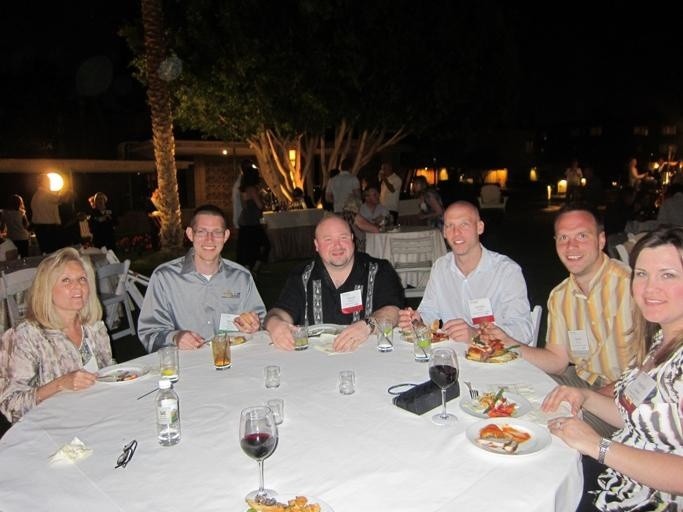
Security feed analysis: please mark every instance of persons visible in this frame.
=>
[399,200,533,345]
[540,225,683,512]
[264,212,405,352]
[0,173,115,258]
[0,247,116,422]
[137,204,267,350]
[478,204,647,439]
[564,156,683,225]
[231,159,445,251]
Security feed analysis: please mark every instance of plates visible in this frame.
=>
[463,351,520,366]
[465,417,552,455]
[95,367,145,384]
[209,333,254,348]
[307,324,340,339]
[459,389,532,418]
[241,495,333,512]
[399,329,449,346]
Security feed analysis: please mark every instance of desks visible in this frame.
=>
[263,207,324,261]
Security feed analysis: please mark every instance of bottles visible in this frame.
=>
[154,378,182,446]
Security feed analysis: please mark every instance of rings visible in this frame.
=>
[556,420,561,427]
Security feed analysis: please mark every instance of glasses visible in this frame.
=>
[192,229,225,238]
[115,440,137,470]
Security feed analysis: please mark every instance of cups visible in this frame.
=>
[376,318,393,352]
[239,406,280,502]
[290,324,308,351]
[339,371,355,395]
[264,399,284,427]
[264,365,280,389]
[157,341,179,380]
[210,335,231,371]
[412,328,431,362]
[429,347,459,426]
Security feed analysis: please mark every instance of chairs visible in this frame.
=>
[94,258,136,343]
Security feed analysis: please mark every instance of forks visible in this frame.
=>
[464,381,478,400]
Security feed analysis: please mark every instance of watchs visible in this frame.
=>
[361,317,375,334]
[597,436,612,465]
